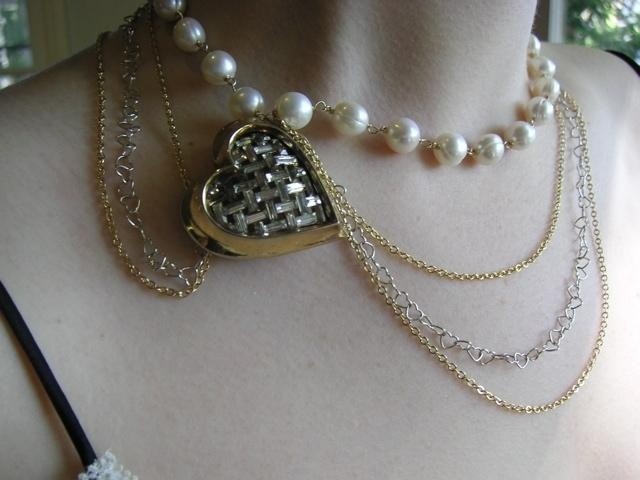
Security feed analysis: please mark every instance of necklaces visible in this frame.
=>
[95,1,610,413]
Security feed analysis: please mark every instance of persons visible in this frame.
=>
[0,0,640,480]
